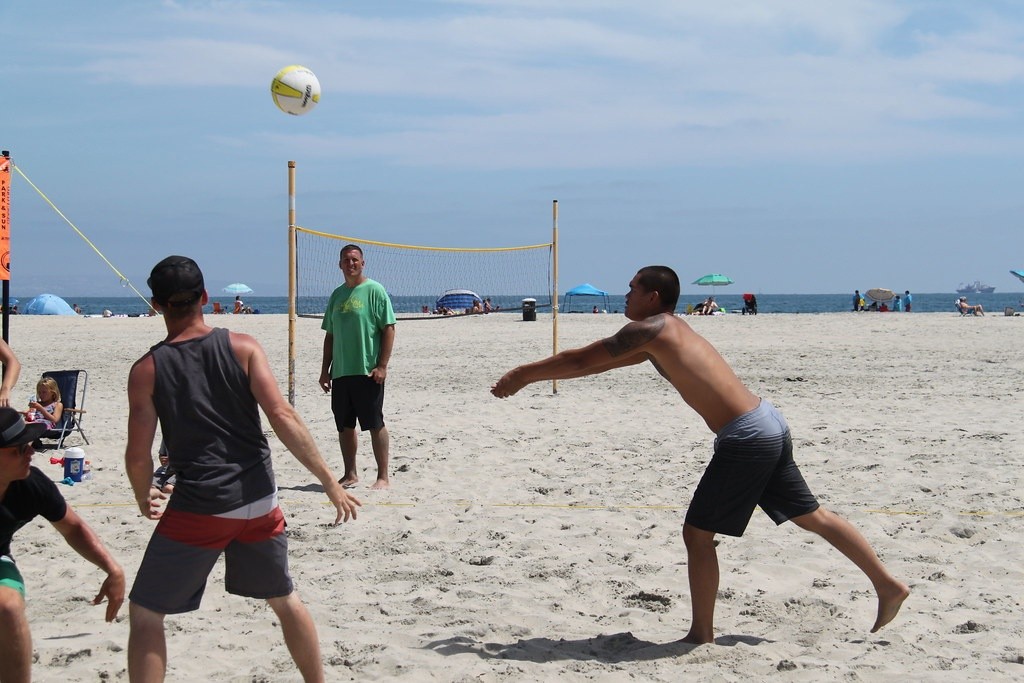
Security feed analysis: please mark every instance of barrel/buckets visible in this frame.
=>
[64,448,86,482]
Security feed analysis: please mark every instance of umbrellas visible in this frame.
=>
[1010,270,1024,282]
[865,288,896,303]
[222,283,253,296]
[691,273,734,301]
[9,297,20,306]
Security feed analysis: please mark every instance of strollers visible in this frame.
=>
[742,294,757,315]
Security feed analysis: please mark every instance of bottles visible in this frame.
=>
[83,461,90,474]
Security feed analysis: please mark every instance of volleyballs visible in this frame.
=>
[270,65,321,117]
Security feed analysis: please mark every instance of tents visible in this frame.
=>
[24,294,76,315]
[562,283,611,313]
[435,289,482,310]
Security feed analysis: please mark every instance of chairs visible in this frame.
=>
[25,370,90,450]
[213,302,224,313]
[741,293,758,315]
[422,305,428,312]
[954,301,976,317]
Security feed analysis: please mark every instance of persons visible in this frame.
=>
[0,407,125,683]
[853,290,865,311]
[319,244,396,490]
[0,305,18,314]
[960,296,983,316]
[483,298,493,314]
[893,295,902,311]
[24,377,64,449]
[73,303,80,313]
[126,256,361,683]
[0,336,21,408]
[235,296,247,313]
[593,306,598,313]
[492,266,910,644]
[471,299,483,313]
[903,290,912,311]
[700,297,718,315]
[102,307,113,317]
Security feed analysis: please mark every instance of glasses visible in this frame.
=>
[0,443,28,456]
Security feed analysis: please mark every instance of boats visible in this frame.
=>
[956,281,996,293]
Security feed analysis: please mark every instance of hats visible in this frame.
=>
[147,254,204,307]
[0,407,47,448]
